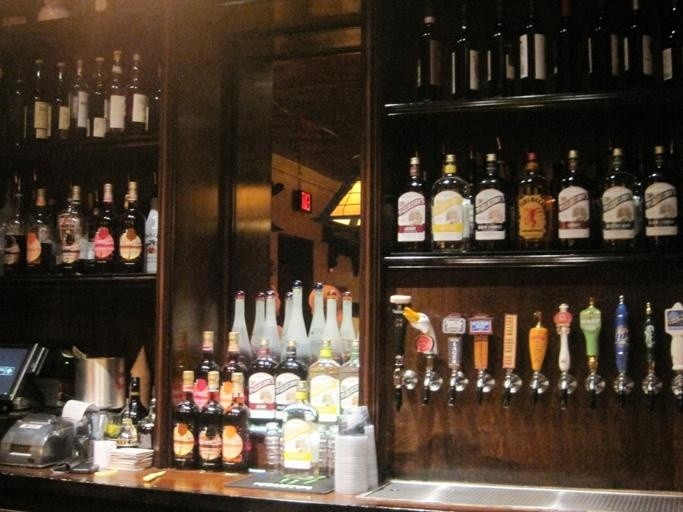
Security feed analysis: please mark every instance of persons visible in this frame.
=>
[306,283,343,329]
[267,283,284,342]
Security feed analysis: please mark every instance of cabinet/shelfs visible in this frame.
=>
[362,1,682,496]
[0,0,226,469]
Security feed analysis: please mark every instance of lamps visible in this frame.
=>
[326,176,360,228]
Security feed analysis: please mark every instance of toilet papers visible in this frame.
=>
[365,425,378,490]
[335,435,369,494]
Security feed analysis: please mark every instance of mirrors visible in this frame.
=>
[226,14,363,367]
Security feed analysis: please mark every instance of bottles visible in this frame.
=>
[221,279,358,344]
[171,371,252,472]
[2,166,158,279]
[9,43,148,146]
[115,379,157,448]
[393,145,676,253]
[191,330,357,424]
[283,381,317,478]
[402,7,676,94]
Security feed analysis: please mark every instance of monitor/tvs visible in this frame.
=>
[0,341,48,401]
